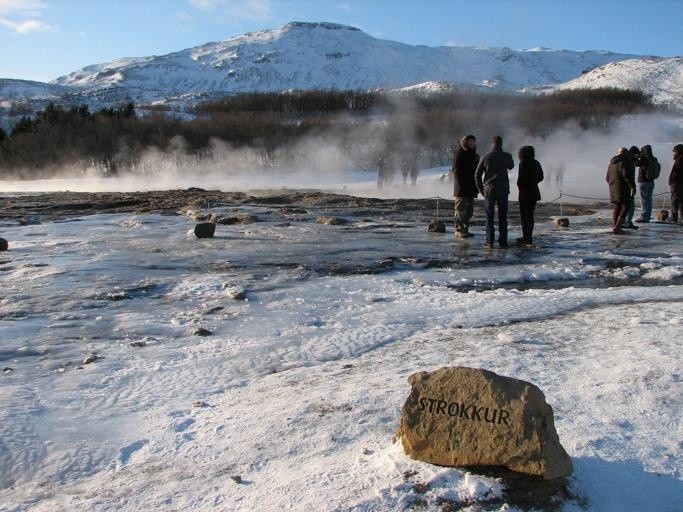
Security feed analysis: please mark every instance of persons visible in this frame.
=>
[606,144,683,234]
[452,135,543,248]
[377,155,419,190]
[548,162,565,184]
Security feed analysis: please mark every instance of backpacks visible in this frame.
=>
[643,156,660,180]
